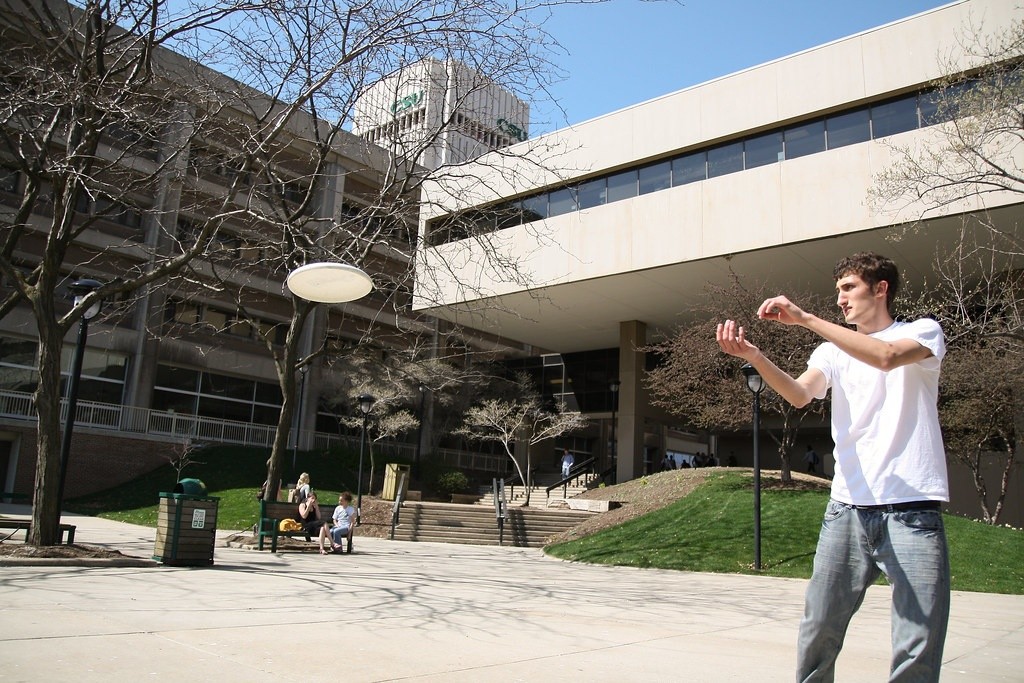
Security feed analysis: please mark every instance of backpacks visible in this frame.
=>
[288,484,307,504]
[257,482,267,502]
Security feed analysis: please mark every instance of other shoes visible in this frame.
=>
[331,542,341,549]
[332,549,343,554]
[319,549,328,555]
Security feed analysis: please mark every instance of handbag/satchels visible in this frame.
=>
[661,458,665,469]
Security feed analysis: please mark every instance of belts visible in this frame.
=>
[855,500,942,512]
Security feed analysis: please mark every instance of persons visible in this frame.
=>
[715,251,951,683]
[295,472,312,542]
[258,457,283,503]
[801,444,822,473]
[298,489,342,555]
[659,451,737,471]
[329,493,357,553]
[560,446,575,480]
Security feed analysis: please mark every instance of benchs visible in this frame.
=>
[451,494,483,503]
[0,519,77,545]
[257,499,354,553]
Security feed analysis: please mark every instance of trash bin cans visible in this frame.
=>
[151,478,221,568]
[382,463,411,502]
